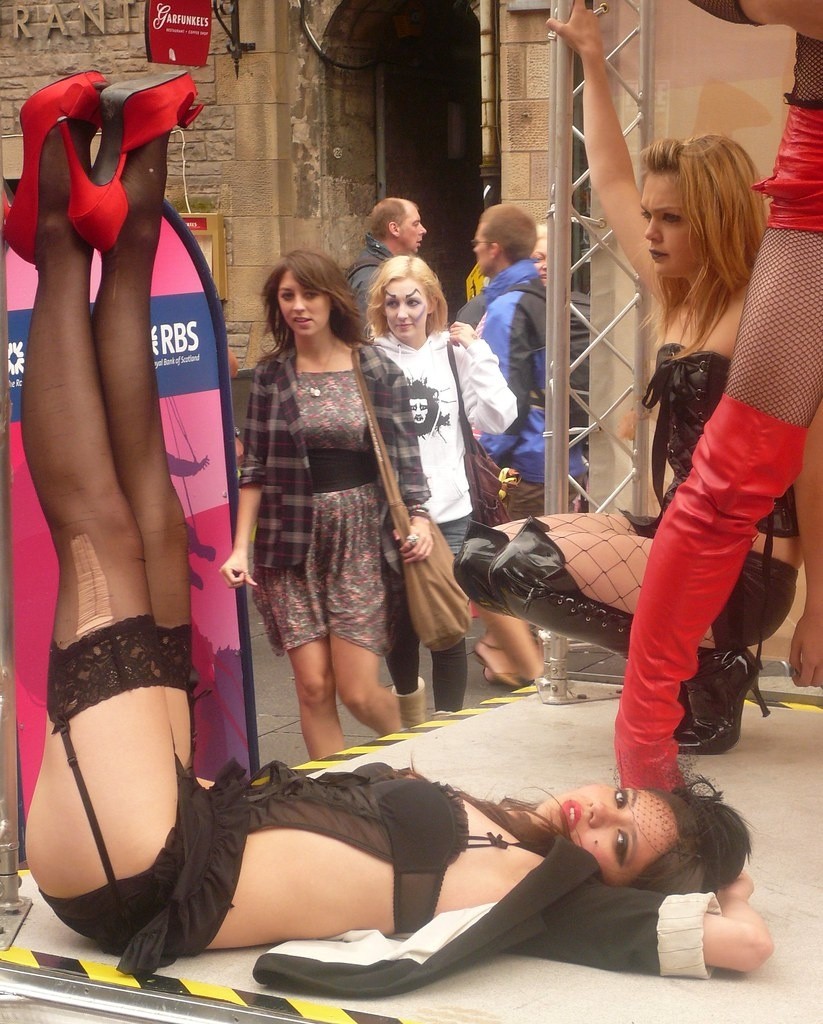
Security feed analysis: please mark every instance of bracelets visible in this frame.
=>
[408,504,431,521]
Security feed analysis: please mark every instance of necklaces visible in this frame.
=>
[299,338,336,398]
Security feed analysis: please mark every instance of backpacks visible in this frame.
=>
[497,281,590,456]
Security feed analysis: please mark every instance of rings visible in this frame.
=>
[789,669,801,677]
[406,534,419,545]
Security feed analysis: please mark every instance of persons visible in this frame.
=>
[453,0,823,754]
[344,197,426,337]
[455,204,590,688]
[455,225,547,330]
[613,0,823,799]
[218,250,434,761]
[367,254,519,727]
[0,71,773,1001]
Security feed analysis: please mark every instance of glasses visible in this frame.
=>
[471,240,499,247]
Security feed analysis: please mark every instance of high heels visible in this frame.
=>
[0,72,110,265]
[58,70,204,251]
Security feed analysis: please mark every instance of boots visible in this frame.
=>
[393,676,426,731]
[488,515,769,755]
[451,519,691,734]
[614,394,808,793]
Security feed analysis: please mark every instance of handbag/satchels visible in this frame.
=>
[463,439,520,527]
[400,513,471,652]
